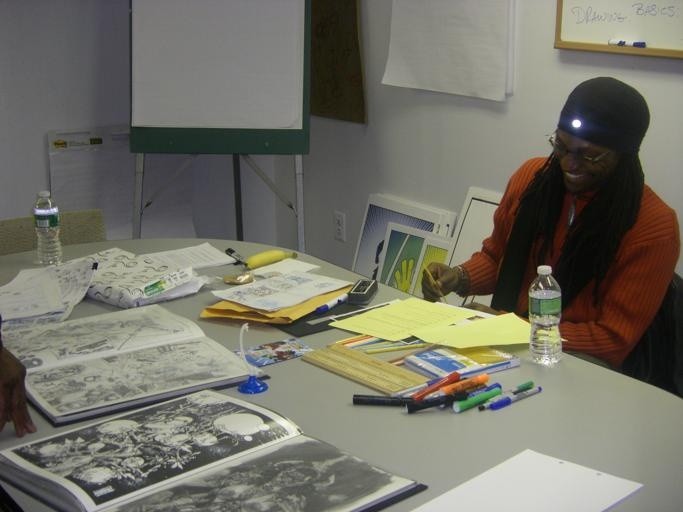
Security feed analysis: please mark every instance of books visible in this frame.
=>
[0,304,253,427]
[0,390,429,512]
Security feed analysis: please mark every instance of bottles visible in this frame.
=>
[32,191,62,265]
[527,265,563,365]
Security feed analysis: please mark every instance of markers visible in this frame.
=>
[225,248,247,265]
[316,292,350,314]
[608,38,645,48]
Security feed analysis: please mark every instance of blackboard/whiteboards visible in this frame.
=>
[129,0,311,154]
[554,0,683,61]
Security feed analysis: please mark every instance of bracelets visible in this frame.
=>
[455,265,467,295]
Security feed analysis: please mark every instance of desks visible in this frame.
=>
[0,236,682,512]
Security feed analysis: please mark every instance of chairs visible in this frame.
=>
[619,270,682,398]
[0,208,108,257]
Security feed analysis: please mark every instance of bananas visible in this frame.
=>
[243,249,298,270]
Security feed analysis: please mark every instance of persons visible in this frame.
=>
[0,315,37,439]
[420,75,681,373]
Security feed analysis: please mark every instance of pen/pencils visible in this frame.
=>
[424,267,449,304]
[353,371,542,414]
[365,343,428,354]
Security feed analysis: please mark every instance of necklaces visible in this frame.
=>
[565,198,578,232]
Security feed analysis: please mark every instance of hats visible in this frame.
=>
[556,77,650,151]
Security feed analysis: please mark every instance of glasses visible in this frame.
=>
[546,131,608,163]
